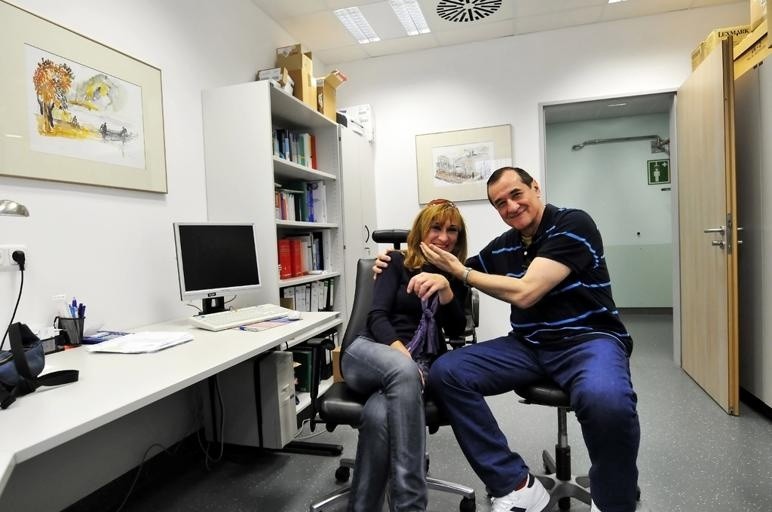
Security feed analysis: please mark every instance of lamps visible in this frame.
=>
[0,199,29,217]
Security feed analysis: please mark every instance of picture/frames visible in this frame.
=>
[415,124,513,206]
[0,0,168,195]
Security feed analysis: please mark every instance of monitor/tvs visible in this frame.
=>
[172,220,264,315]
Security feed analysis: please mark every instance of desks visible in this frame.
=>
[1,300,338,495]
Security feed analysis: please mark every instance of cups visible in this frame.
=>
[53,316,86,346]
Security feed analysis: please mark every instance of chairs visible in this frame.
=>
[310,230,476,512]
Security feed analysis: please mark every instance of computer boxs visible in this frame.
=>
[200,349,298,450]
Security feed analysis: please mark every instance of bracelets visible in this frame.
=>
[463,267,472,288]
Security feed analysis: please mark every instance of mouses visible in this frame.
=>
[285,309,303,321]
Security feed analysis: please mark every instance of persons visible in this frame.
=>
[373,167,640,512]
[340,198,467,512]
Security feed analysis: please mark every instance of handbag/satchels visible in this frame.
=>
[0,322,79,409]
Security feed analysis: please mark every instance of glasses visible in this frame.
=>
[428,199,456,207]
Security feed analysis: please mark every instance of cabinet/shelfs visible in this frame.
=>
[202,81,347,416]
[338,125,377,325]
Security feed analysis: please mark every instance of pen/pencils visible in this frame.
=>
[78,302,83,317]
[68,304,73,318]
[72,297,78,319]
[81,306,85,318]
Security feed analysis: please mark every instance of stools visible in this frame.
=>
[515,386,592,512]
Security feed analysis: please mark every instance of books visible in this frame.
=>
[272,129,339,380]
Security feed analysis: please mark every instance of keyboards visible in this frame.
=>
[187,302,292,333]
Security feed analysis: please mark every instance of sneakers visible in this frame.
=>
[490,471,550,512]
[590,498,601,512]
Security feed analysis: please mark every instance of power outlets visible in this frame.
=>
[0,245,30,272]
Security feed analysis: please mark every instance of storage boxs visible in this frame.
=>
[692,0,767,75]
[332,346,345,383]
[275,43,347,122]
